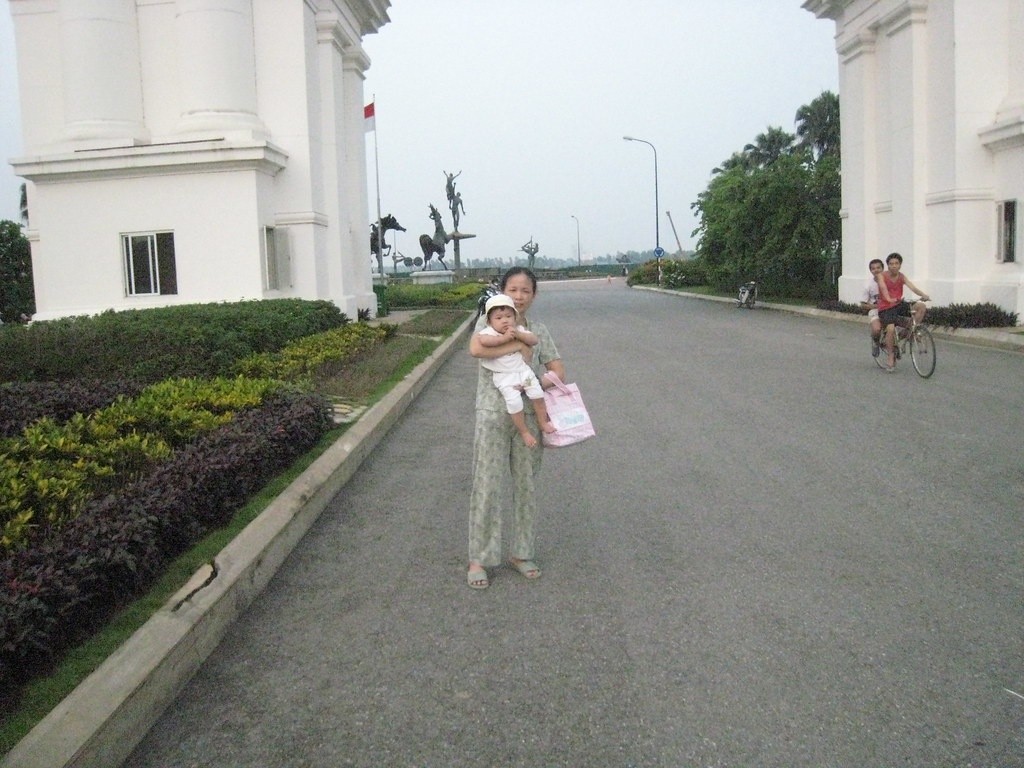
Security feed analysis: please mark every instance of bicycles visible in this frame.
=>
[874,296,937,379]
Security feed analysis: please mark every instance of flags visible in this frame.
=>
[364,102,375,132]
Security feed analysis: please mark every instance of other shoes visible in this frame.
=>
[888,353,896,368]
[898,329,915,342]
[871,336,880,357]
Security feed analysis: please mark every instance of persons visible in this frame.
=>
[878,252,929,372]
[443,170,465,232]
[859,258,900,360]
[392,253,404,263]
[469,266,565,590]
[478,295,557,448]
[522,242,539,268]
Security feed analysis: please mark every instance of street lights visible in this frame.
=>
[623,135,660,288]
[571,215,580,270]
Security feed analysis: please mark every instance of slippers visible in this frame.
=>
[467,566,489,589]
[506,558,542,579]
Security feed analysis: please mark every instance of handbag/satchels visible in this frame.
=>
[540,371,595,448]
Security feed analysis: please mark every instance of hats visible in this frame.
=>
[485,295,520,325]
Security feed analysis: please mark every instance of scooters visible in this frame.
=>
[736,274,763,310]
